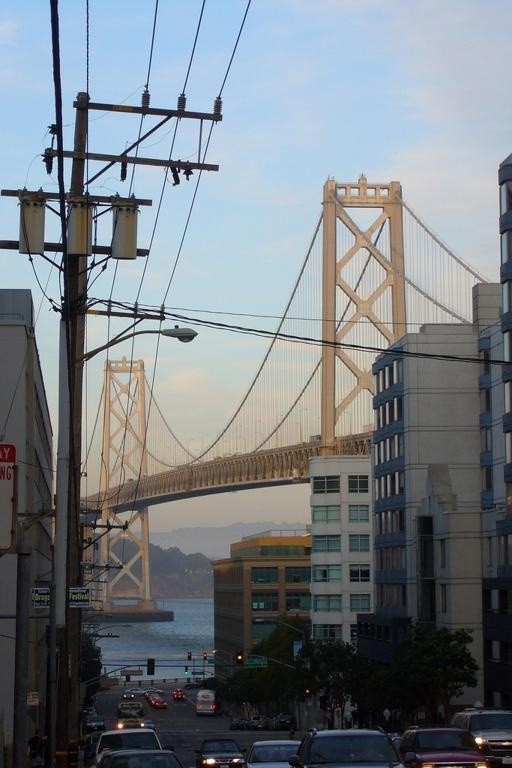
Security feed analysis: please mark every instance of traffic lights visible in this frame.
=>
[184,651,243,672]
[147,658,155,675]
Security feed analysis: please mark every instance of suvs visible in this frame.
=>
[450,709,512,766]
[289,725,405,768]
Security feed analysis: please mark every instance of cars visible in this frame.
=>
[245,739,302,768]
[117,683,217,729]
[95,727,183,768]
[193,738,248,768]
[399,727,491,768]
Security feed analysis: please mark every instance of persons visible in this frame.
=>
[381,705,391,720]
[438,701,446,727]
[27,727,41,757]
